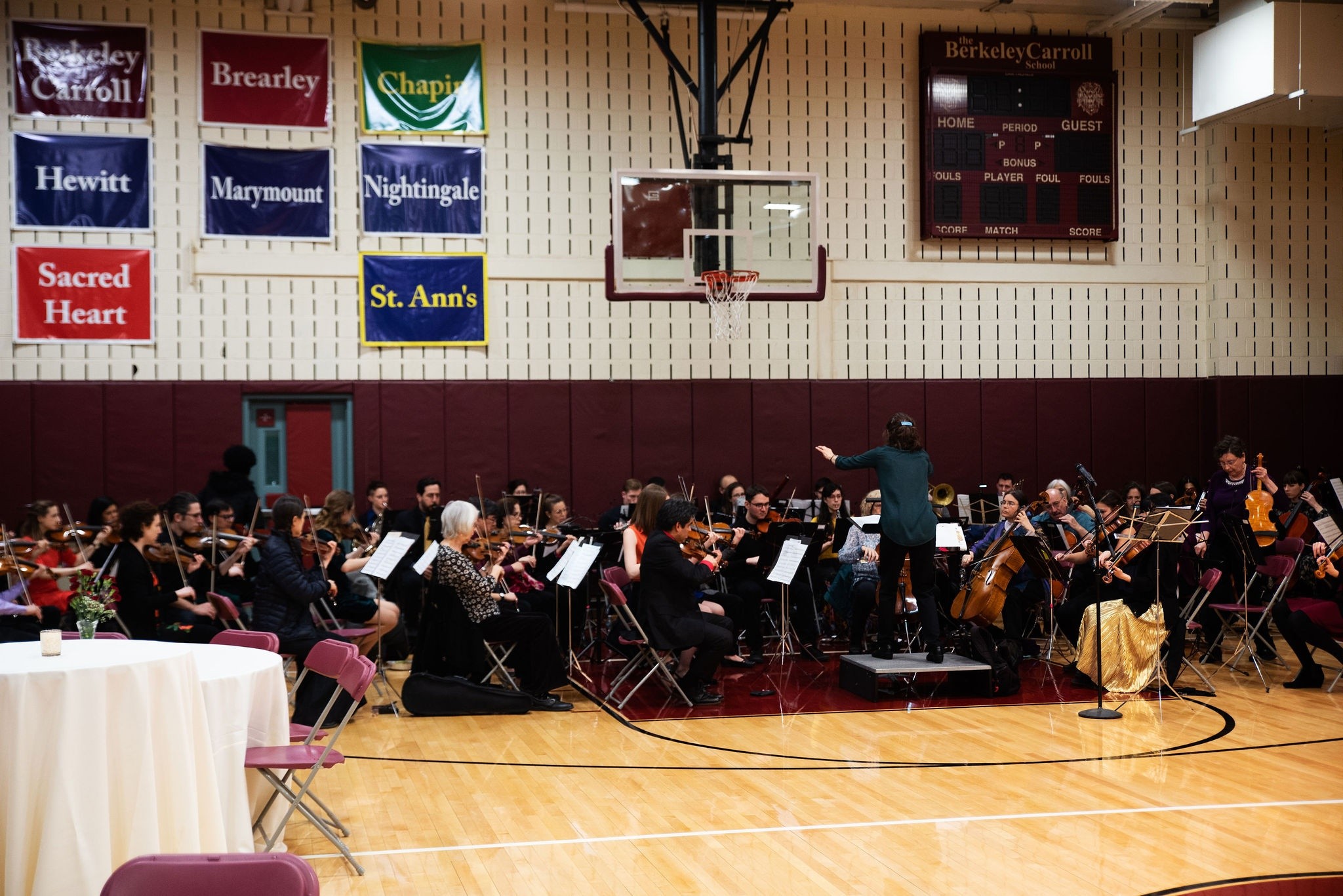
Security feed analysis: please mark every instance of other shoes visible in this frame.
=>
[720,655,755,668]
[707,679,717,684]
[1070,676,1092,685]
[992,667,1021,697]
[751,647,762,663]
[800,645,828,660]
[1248,648,1279,661]
[848,629,862,654]
[318,721,337,728]
[1063,661,1079,672]
[1200,651,1222,664]
[1283,664,1324,688]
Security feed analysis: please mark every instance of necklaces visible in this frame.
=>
[1225,478,1244,486]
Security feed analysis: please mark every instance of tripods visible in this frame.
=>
[1201,520,1270,696]
[1111,507,1194,724]
[343,523,1076,707]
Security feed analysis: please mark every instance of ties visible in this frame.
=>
[423,517,432,553]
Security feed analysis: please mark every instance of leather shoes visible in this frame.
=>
[519,688,573,711]
[672,683,725,704]
[927,646,943,663]
[872,644,893,660]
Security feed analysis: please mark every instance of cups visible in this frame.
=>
[40,629,62,656]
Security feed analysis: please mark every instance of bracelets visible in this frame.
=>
[830,455,835,462]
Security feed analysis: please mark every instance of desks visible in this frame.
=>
[0,637,297,896]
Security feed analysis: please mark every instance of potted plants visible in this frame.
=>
[69,567,118,638]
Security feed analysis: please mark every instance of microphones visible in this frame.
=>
[1075,462,1098,487]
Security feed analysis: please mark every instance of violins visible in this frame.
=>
[0,554,62,582]
[334,523,372,544]
[1244,453,1277,547]
[297,534,341,557]
[756,511,803,533]
[688,521,761,543]
[1102,541,1154,584]
[184,526,264,551]
[0,538,69,555]
[519,524,568,546]
[466,537,515,560]
[488,527,538,544]
[46,520,123,543]
[1086,518,1127,559]
[1314,539,1343,579]
[144,542,218,571]
[680,539,728,568]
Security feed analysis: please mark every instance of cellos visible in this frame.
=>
[950,491,1050,624]
[875,542,920,655]
[1037,491,1085,604]
[1276,472,1323,538]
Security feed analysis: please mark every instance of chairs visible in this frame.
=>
[1255,535,1304,636]
[599,565,668,687]
[1201,554,1295,695]
[208,590,294,683]
[287,636,360,743]
[1149,566,1222,692]
[210,623,280,648]
[245,655,383,876]
[311,597,377,647]
[99,850,322,896]
[1031,548,1077,661]
[738,597,792,657]
[480,638,522,694]
[600,577,693,710]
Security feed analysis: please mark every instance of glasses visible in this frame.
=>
[485,518,496,524]
[550,506,567,515]
[1219,456,1239,466]
[217,514,234,519]
[510,514,523,520]
[1001,501,1019,507]
[181,513,200,518]
[1045,495,1064,510]
[749,503,770,509]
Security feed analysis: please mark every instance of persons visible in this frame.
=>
[435,498,574,712]
[1192,433,1343,688]
[598,478,756,704]
[198,443,266,530]
[20,498,120,633]
[928,472,1100,696]
[0,563,49,643]
[807,477,882,655]
[816,412,947,664]
[144,490,262,631]
[312,488,400,657]
[1053,479,1224,692]
[461,480,592,670]
[114,500,222,643]
[254,496,351,729]
[358,479,446,654]
[704,474,831,665]
[86,497,124,573]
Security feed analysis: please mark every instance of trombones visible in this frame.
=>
[926,481,955,518]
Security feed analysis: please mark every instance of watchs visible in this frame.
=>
[91,543,99,550]
